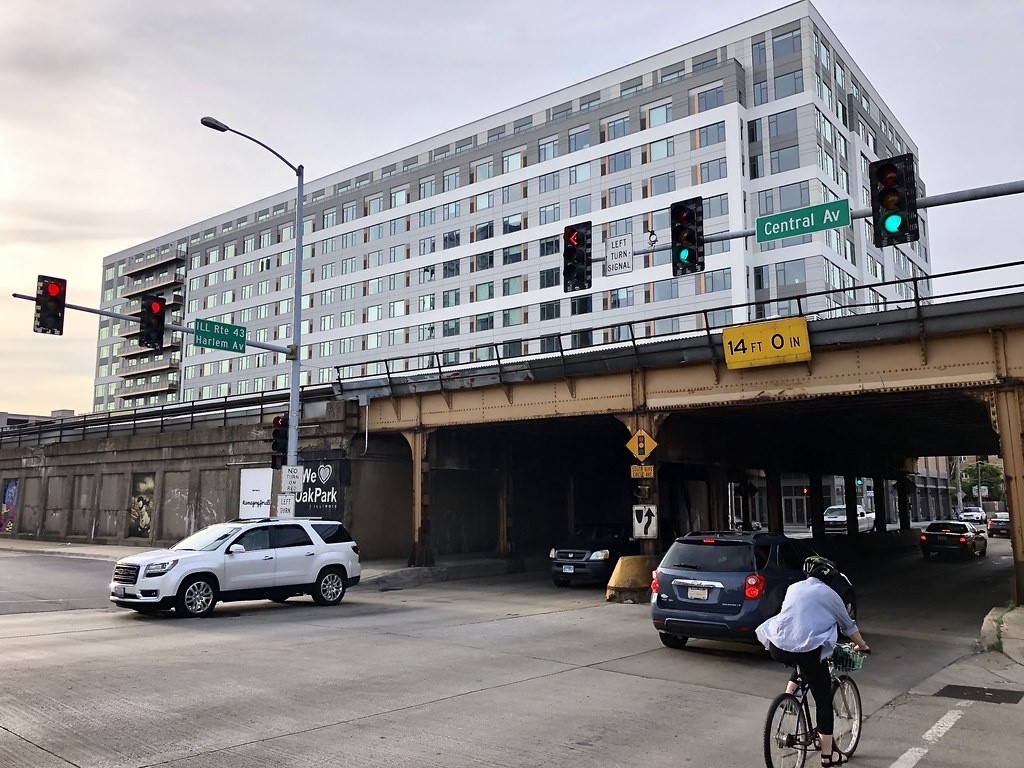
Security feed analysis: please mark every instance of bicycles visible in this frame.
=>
[763,641,872,768]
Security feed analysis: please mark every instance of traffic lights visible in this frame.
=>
[671,195,705,278]
[270,411,288,470]
[803,489,808,494]
[139,294,166,350]
[563,219,594,293]
[857,477,862,486]
[32,274,67,337]
[868,152,920,249]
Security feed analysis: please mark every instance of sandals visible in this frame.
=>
[779,699,796,716]
[821,751,849,767]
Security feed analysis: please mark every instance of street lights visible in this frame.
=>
[198,118,304,516]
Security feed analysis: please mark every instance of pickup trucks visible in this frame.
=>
[823,504,877,537]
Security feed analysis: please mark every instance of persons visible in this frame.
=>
[755,557,869,768]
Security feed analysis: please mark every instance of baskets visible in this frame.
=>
[831,644,867,674]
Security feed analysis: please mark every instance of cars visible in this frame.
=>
[920,520,987,562]
[958,507,987,525]
[986,512,1011,538]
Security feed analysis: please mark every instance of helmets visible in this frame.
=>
[802,555,836,579]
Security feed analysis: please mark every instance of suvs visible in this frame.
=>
[548,517,679,588]
[107,517,362,618]
[651,528,852,649]
